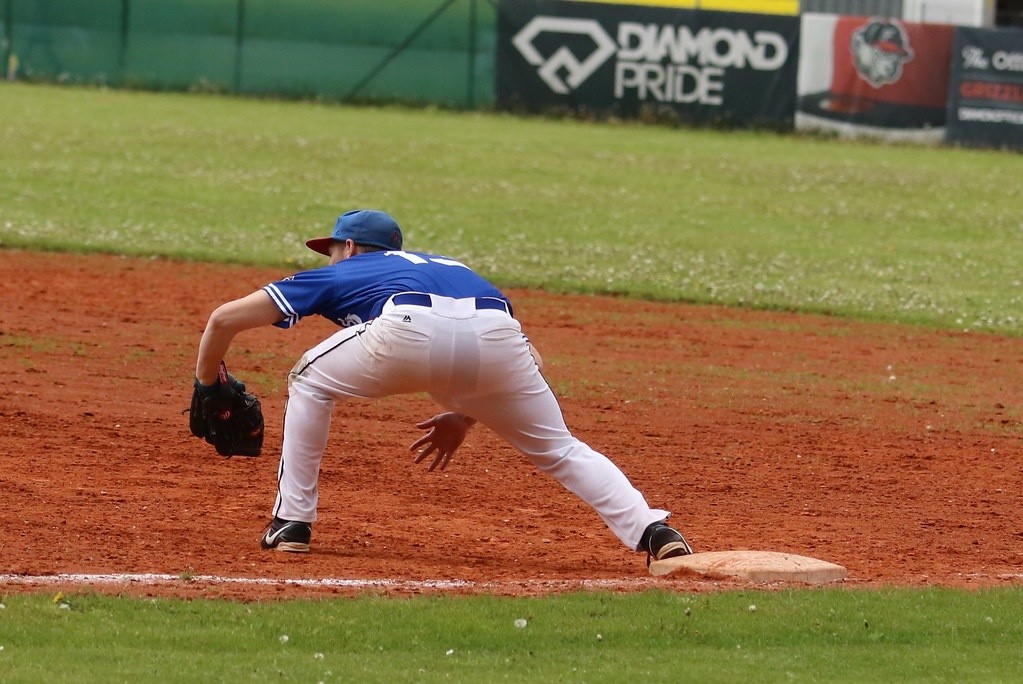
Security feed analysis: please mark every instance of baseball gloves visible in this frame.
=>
[190,375,265,457]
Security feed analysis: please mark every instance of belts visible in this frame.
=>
[392,293,506,311]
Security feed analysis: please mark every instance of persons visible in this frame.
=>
[189,209,693,559]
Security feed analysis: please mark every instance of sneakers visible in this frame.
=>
[255,517,312,552]
[640,517,692,568]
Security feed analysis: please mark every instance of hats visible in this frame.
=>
[305,210,403,256]
